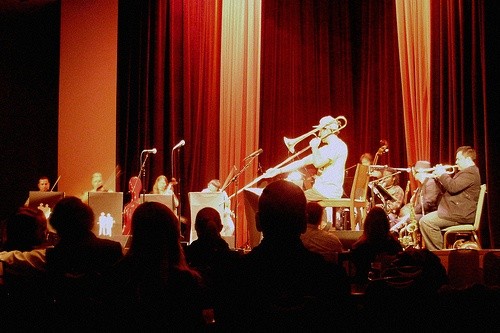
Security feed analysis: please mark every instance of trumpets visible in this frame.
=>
[411,165,459,178]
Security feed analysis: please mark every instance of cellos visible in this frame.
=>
[368,140,389,176]
[166,178,178,195]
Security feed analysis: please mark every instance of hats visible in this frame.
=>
[415,161,432,168]
[49,197,94,230]
[312,115,338,130]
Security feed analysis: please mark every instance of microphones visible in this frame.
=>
[173,139,186,151]
[244,148,264,161]
[142,148,157,154]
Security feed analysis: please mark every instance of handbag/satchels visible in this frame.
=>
[367,246,448,290]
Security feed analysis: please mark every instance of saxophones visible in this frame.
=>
[406,186,420,246]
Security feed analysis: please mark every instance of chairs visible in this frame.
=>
[440,183,487,251]
[317,163,370,232]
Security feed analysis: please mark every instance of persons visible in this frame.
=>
[81,171,113,205]
[262,115,348,205]
[200,179,235,236]
[389,160,441,234]
[381,167,403,225]
[403,145,481,251]
[24,177,51,207]
[360,153,374,165]
[0,181,499,333]
[149,175,179,215]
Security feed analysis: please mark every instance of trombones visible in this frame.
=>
[218,116,347,207]
